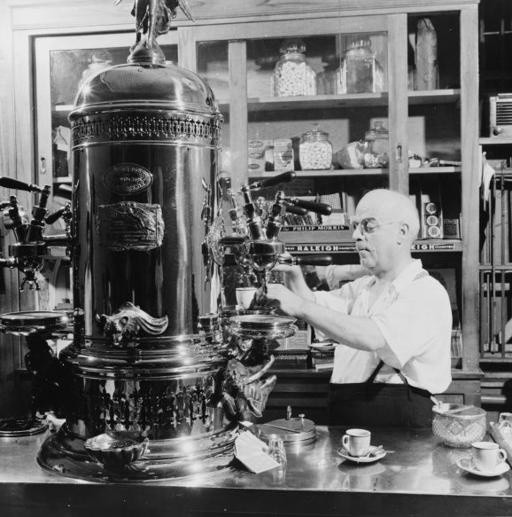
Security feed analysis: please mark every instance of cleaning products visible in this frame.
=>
[415,18,436,93]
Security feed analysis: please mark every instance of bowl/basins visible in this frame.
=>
[431,403,488,449]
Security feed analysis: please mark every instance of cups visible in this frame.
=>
[471,442,508,471]
[235,288,258,311]
[341,429,373,457]
[266,438,287,468]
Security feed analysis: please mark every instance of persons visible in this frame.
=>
[255,188,453,428]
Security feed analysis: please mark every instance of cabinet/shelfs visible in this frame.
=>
[0,0,512,423]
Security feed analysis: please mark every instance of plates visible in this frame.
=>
[337,445,388,463]
[455,456,511,478]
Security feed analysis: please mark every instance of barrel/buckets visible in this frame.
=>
[0,309,73,416]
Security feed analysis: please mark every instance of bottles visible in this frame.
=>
[271,37,317,98]
[360,120,390,169]
[297,121,334,172]
[332,36,387,96]
